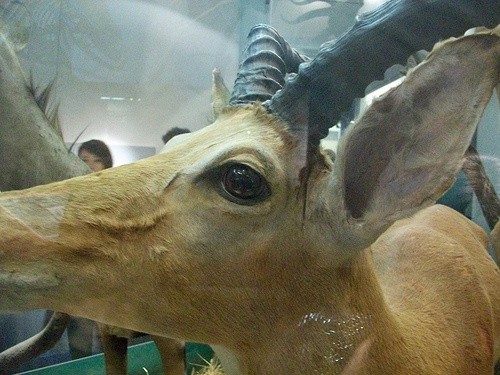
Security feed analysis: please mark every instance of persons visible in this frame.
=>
[65,131,123,358]
[92,317,191,373]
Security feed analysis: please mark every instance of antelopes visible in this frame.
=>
[1,0,500,375]
